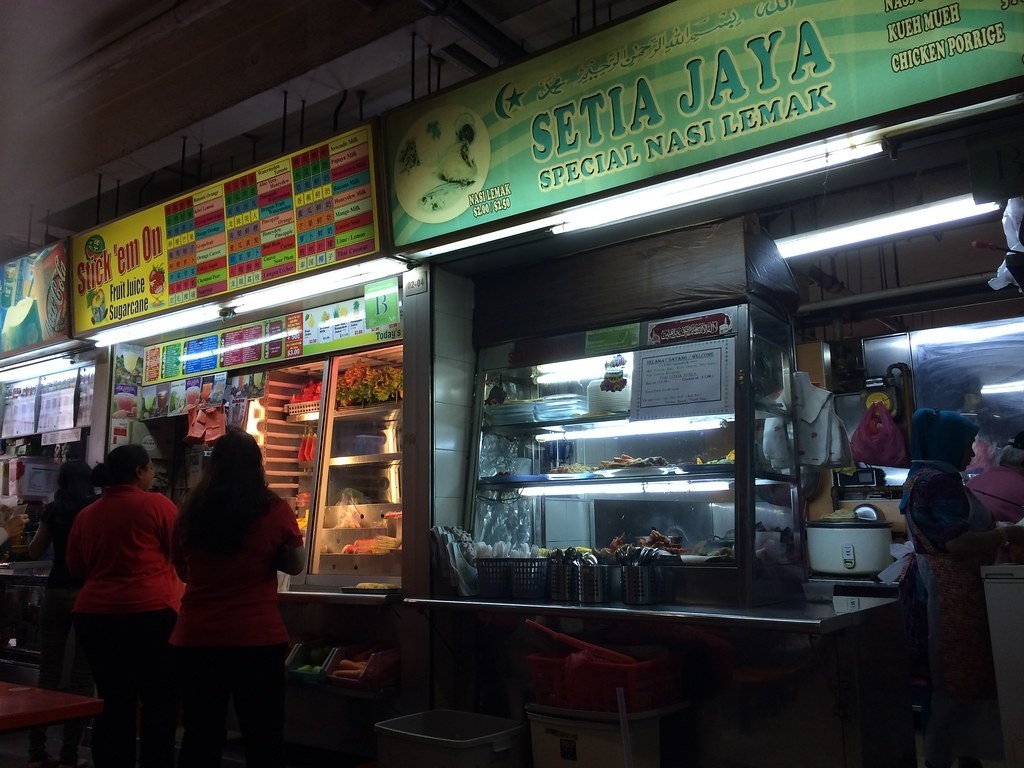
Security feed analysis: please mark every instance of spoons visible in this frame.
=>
[555,546,660,564]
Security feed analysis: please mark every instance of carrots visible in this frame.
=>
[332,659,367,679]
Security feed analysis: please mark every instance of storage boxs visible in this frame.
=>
[284,634,399,759]
[527,712,661,768]
[374,707,526,768]
[526,650,676,712]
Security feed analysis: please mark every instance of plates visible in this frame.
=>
[592,465,676,478]
[480,474,546,484]
[533,392,585,422]
[393,104,491,223]
[585,378,632,415]
[678,462,735,474]
[483,400,533,426]
[545,471,593,481]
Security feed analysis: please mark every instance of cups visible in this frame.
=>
[117,392,134,410]
[187,373,262,403]
[158,391,168,410]
[124,353,138,371]
[144,395,153,410]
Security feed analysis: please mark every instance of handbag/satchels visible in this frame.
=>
[851,402,910,468]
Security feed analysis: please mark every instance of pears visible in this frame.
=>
[296,665,322,672]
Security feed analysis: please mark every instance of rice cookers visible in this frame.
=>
[805,503,896,576]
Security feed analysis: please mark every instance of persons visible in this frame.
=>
[28,459,96,768]
[898,408,1024,768]
[169,429,304,768]
[0,504,30,546]
[67,444,184,768]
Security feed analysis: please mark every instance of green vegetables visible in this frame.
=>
[337,358,404,407]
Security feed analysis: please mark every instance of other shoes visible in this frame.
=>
[25,755,61,768]
[58,758,89,768]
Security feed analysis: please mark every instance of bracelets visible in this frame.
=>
[995,527,1010,548]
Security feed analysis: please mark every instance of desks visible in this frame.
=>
[0,681,104,734]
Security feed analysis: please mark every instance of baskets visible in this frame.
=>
[473,557,550,598]
[526,647,685,713]
